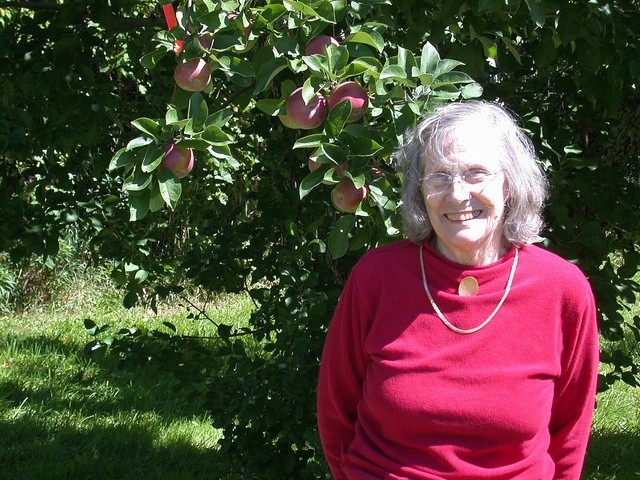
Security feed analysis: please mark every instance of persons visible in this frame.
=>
[316,97,600,480]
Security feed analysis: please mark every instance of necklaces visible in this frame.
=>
[419,244,519,334]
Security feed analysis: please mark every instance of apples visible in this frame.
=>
[156,142,195,179]
[362,168,386,208]
[174,57,211,91]
[286,86,328,128]
[308,147,350,185]
[304,35,340,59]
[330,178,367,214]
[194,32,219,57]
[225,12,251,40]
[327,81,369,123]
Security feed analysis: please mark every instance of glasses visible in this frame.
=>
[418,168,504,191]
